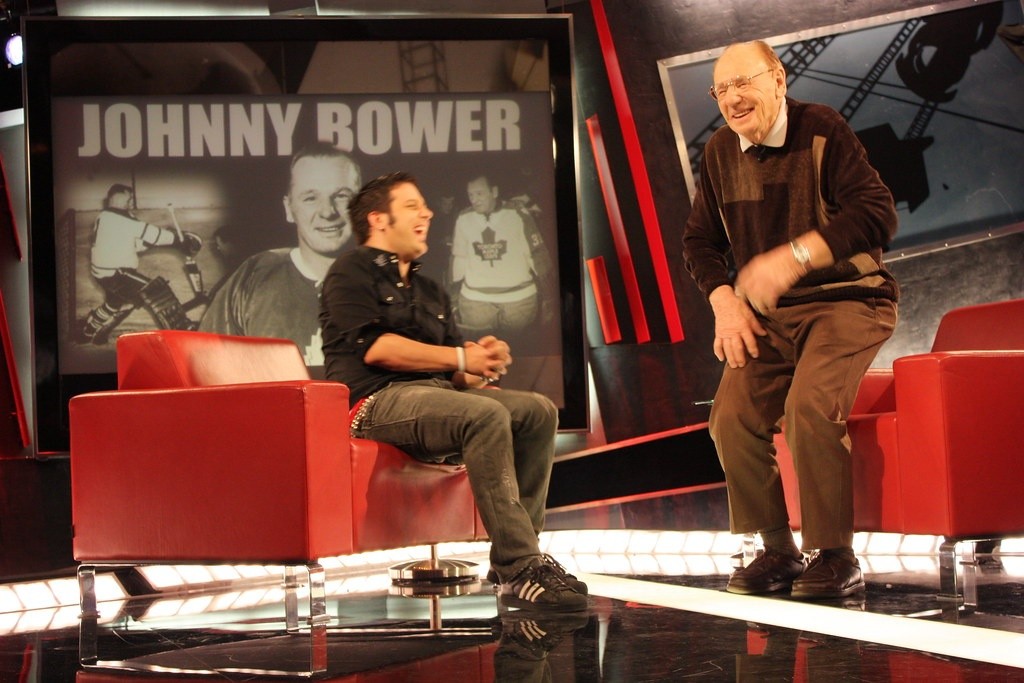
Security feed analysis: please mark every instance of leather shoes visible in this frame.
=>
[791,554,866,598]
[727,554,806,593]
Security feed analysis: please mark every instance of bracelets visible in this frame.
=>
[456,346,465,372]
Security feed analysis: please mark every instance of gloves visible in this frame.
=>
[175,231,202,256]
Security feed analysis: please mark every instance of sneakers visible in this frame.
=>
[501,557,588,612]
[546,561,587,595]
[502,609,588,650]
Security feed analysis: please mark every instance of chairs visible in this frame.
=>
[72,330,496,683]
[746,299,1023,603]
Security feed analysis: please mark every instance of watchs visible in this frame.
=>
[791,239,813,274]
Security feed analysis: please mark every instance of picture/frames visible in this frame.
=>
[17,11,592,457]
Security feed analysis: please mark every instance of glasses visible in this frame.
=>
[708,69,774,101]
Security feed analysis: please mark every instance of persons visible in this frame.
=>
[448,176,553,330]
[492,613,589,683]
[315,172,589,610]
[213,224,234,259]
[682,40,900,599]
[198,142,362,367]
[78,183,200,345]
